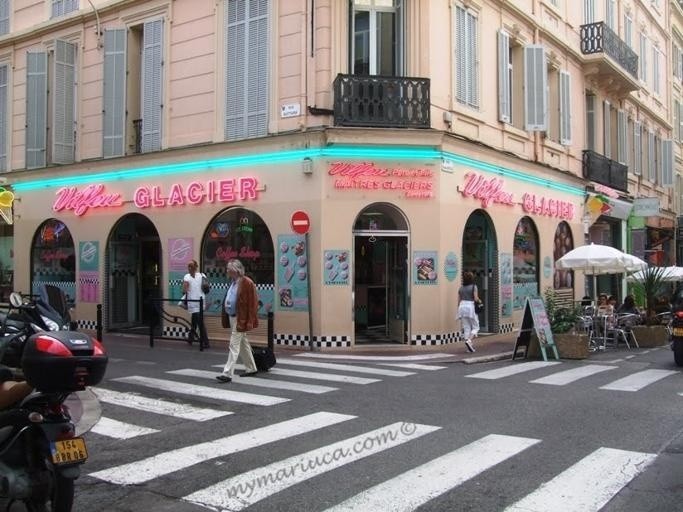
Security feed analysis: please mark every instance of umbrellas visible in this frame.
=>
[553,241,633,304]
[624,265,680,283]
[664,265,682,285]
[583,250,647,305]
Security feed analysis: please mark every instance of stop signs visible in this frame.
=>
[291,210,310,234]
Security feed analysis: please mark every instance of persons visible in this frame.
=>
[215,258,260,384]
[582,295,645,343]
[456,270,482,354]
[180,259,213,352]
[668,281,683,342]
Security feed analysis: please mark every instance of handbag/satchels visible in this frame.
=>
[201,276,210,293]
[475,302,485,313]
[178,294,187,310]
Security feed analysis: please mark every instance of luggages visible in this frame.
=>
[253,348,276,371]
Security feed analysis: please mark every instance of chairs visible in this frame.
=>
[572,304,640,352]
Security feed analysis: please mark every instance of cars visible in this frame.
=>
[667,281,683,365]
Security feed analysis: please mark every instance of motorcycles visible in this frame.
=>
[0,290,111,512]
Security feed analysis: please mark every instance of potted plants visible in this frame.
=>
[543,288,590,359]
[624,259,675,347]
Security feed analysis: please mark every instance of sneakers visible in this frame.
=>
[240,372,257,376]
[216,376,232,382]
[465,339,476,352]
